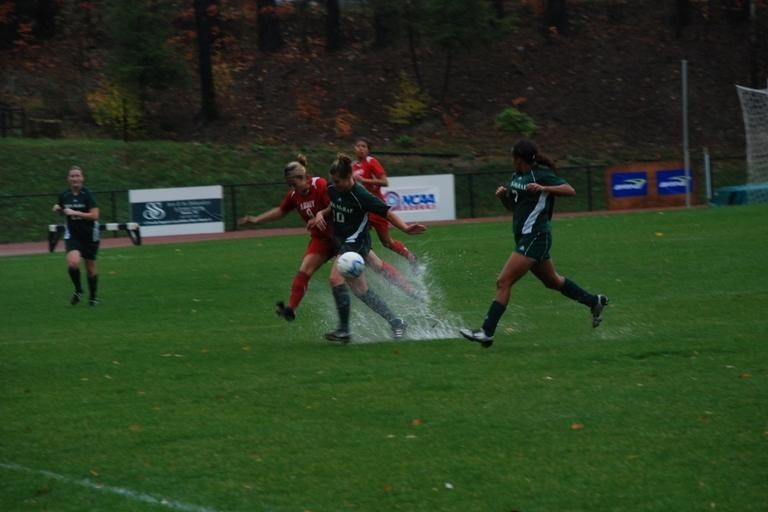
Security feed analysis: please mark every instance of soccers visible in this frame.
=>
[337,252,364,278]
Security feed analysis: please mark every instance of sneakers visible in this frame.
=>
[591,294,609,328]
[391,319,408,341]
[325,328,350,341]
[89,296,98,305]
[459,327,495,348]
[275,301,295,321]
[71,291,82,305]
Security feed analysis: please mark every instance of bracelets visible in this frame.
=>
[539,184,545,192]
[256,215,260,222]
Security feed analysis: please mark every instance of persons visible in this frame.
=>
[52,166,103,307]
[238,154,430,320]
[314,154,426,343]
[460,139,609,347]
[347,138,421,276]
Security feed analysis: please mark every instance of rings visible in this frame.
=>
[318,220,323,223]
